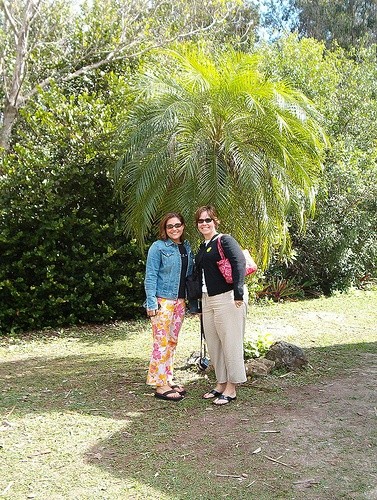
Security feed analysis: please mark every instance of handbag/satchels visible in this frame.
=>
[217,235,257,284]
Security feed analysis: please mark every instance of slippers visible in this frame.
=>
[202,390,223,399]
[154,390,183,401]
[212,395,237,405]
[170,384,186,395]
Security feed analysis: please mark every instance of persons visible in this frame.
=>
[145,212,198,400]
[194,205,257,405]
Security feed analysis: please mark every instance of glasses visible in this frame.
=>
[197,218,213,223]
[166,222,183,229]
